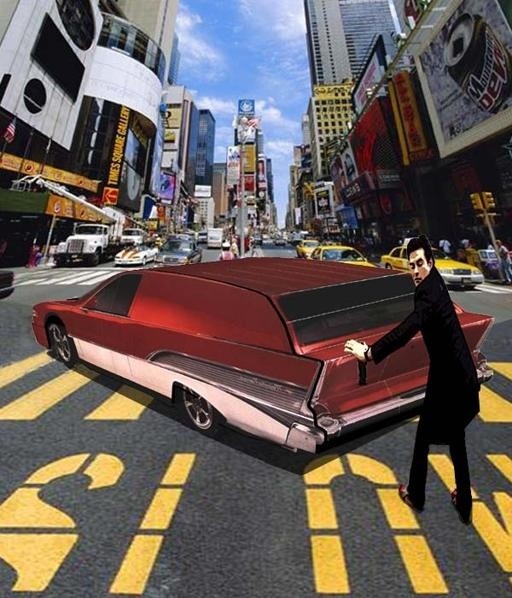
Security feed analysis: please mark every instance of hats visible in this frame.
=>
[222,241,230,247]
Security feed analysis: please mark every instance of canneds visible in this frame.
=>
[443,11,512,115]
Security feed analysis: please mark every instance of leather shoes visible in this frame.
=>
[399,484,425,512]
[451,488,471,525]
